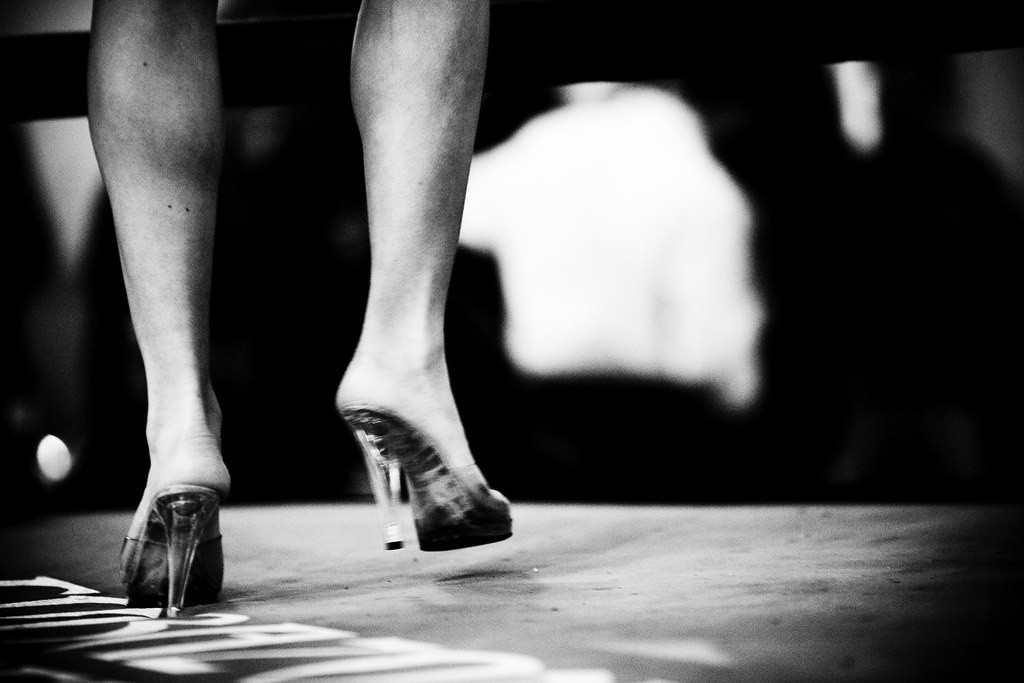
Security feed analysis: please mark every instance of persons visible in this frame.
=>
[87,0,513,616]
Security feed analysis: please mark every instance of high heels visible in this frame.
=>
[342,405,515,556]
[119,485,226,616]
